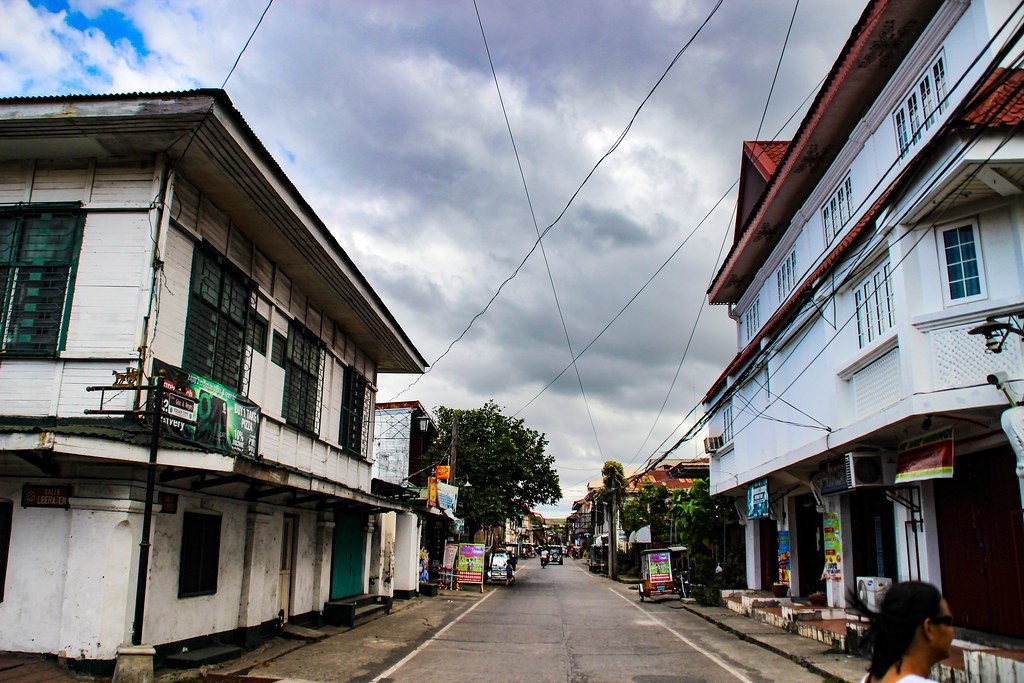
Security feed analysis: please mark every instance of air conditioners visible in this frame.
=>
[857,576,892,613]
[845,449,905,489]
[704,436,724,454]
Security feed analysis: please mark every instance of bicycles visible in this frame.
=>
[542,561,546,569]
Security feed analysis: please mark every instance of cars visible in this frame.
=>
[547,545,563,565]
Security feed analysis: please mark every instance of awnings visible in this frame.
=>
[629,525,652,543]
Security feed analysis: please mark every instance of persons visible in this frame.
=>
[522,545,537,560]
[540,548,549,566]
[564,547,578,559]
[505,560,514,587]
[484,556,491,585]
[845,581,955,683]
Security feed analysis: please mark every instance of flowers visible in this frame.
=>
[772,581,786,585]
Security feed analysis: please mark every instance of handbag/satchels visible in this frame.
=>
[716,563,722,573]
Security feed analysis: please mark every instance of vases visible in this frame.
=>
[808,595,827,607]
[772,585,788,597]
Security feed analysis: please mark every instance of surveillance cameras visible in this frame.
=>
[987,371,1010,385]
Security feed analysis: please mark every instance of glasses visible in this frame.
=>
[927,616,953,627]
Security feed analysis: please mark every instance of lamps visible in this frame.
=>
[417,414,430,431]
